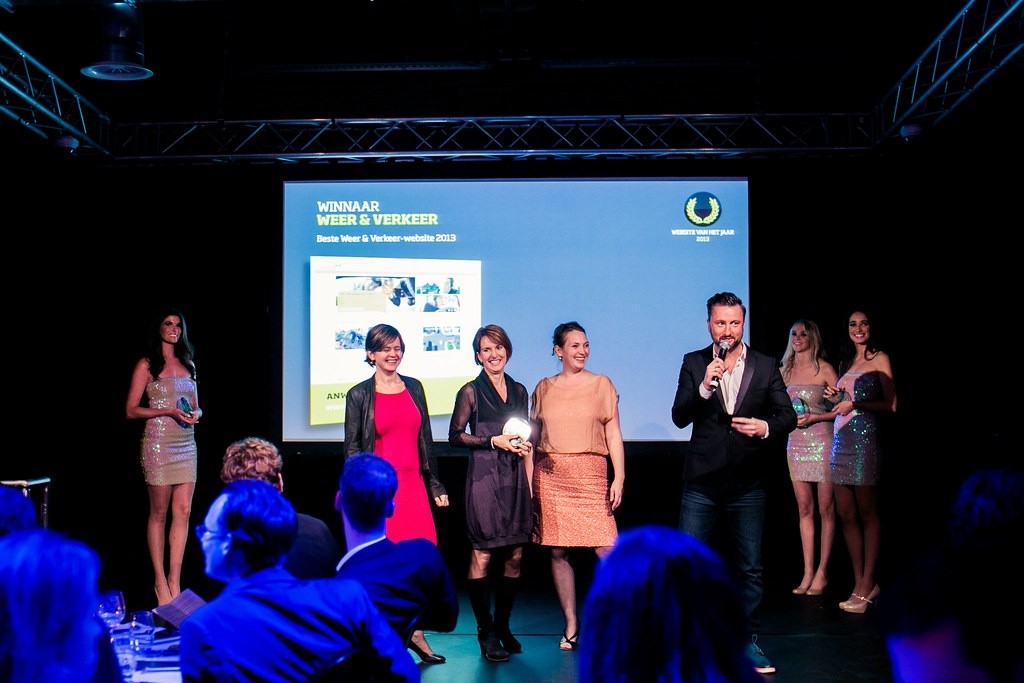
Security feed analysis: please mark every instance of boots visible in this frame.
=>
[494,575,522,652]
[469,575,509,661]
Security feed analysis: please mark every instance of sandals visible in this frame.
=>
[560,624,577,651]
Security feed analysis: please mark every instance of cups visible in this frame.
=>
[131,611,155,651]
[110,636,137,683]
[99,591,126,628]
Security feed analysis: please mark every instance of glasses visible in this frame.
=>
[196,525,224,540]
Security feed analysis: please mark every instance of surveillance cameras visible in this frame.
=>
[899,125,921,143]
[57,137,80,154]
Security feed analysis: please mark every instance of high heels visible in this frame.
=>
[838,584,880,614]
[410,639,446,663]
[793,577,829,596]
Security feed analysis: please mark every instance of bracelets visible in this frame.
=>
[490,436,496,450]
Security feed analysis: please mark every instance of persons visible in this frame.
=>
[447,324,533,662]
[573,524,757,683]
[822,307,898,614]
[887,463,1024,683]
[779,318,840,596]
[344,322,450,663]
[671,292,799,674]
[218,437,336,580]
[0,487,127,683]
[333,453,459,641]
[125,308,203,607]
[179,480,422,683]
[0,529,106,683]
[523,321,625,651]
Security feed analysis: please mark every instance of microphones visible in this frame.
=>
[709,340,731,388]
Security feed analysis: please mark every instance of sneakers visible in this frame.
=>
[746,642,776,673]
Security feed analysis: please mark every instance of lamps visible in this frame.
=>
[79,0,153,81]
